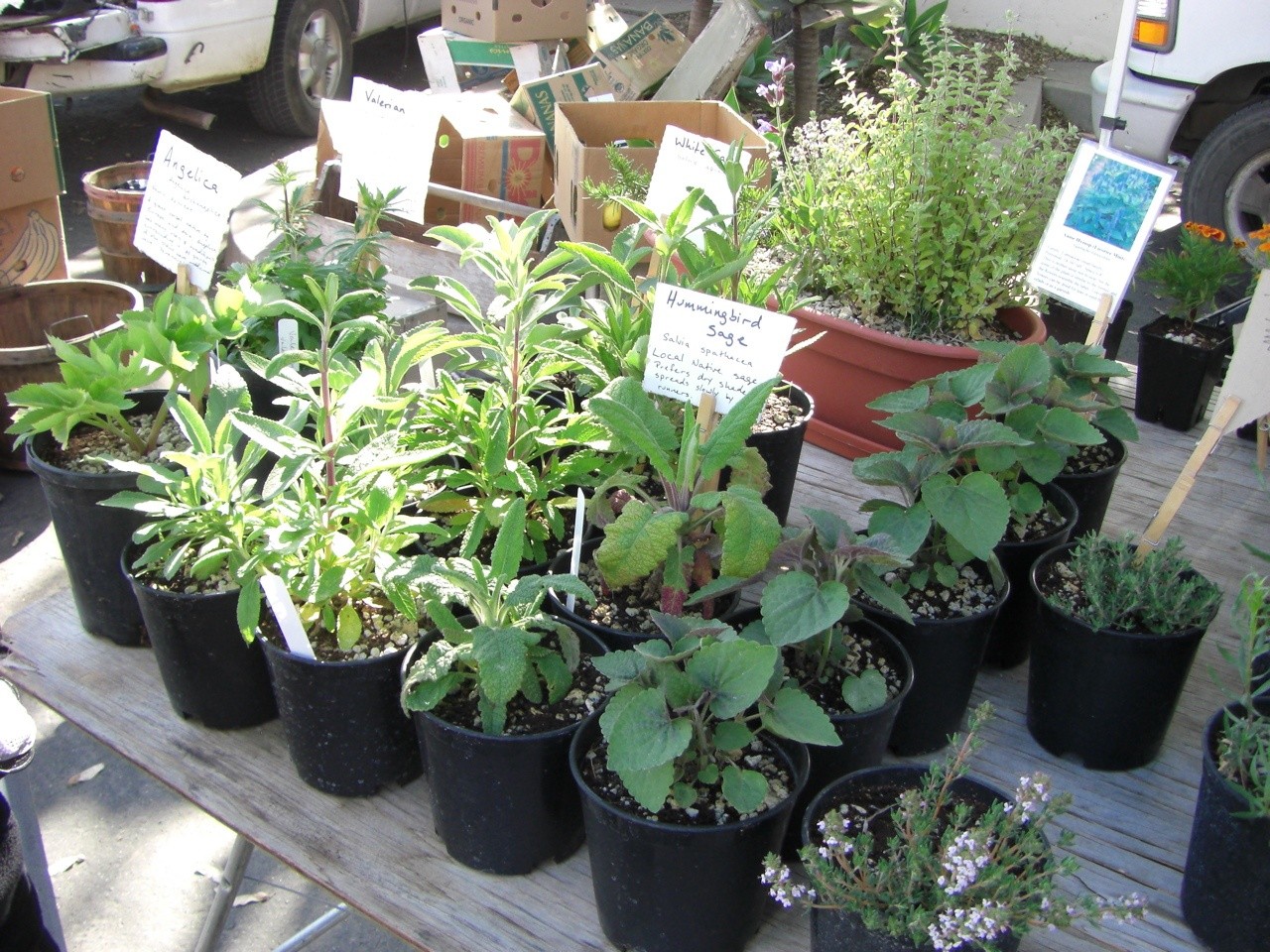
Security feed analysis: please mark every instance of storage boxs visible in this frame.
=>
[317,1,770,262]
[0,195,71,288]
[0,86,67,210]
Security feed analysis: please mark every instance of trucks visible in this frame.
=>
[1083,1,1268,308]
[1,0,442,140]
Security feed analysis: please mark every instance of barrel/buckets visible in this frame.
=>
[79,161,176,293]
[0,278,144,471]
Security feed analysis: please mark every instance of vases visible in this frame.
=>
[802,764,1054,951]
[1135,315,1232,432]
[642,225,1048,463]
[1041,297,1134,387]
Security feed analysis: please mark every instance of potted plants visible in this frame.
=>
[4,133,1269,952]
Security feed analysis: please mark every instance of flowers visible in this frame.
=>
[755,8,1080,343]
[758,700,1148,952]
[1245,223,1270,298]
[1137,223,1247,336]
